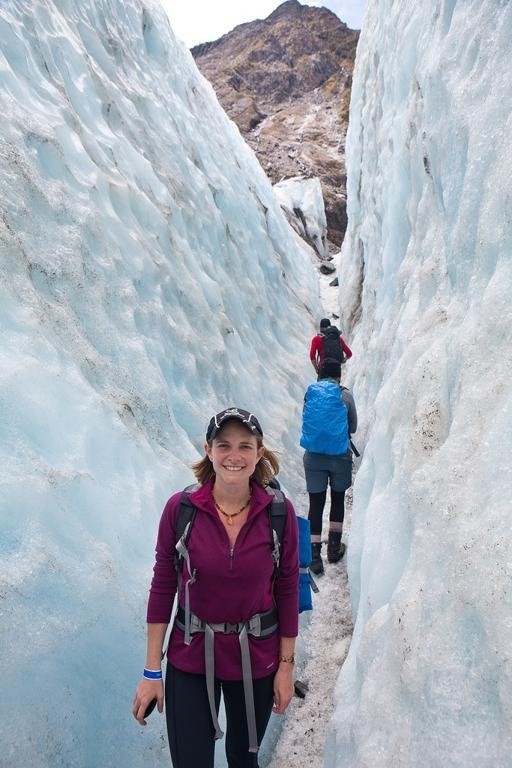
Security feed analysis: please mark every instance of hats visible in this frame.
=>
[205,407,265,449]
[319,317,332,332]
[319,357,342,378]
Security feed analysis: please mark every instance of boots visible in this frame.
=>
[309,540,325,576]
[326,530,346,563]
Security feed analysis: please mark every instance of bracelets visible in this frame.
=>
[140,665,164,682]
[277,652,297,666]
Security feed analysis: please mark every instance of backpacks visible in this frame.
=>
[298,379,351,457]
[317,329,345,363]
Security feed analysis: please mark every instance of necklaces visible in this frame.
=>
[209,494,256,526]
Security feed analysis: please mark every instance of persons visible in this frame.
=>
[128,403,305,768]
[308,317,355,386]
[296,357,359,578]
